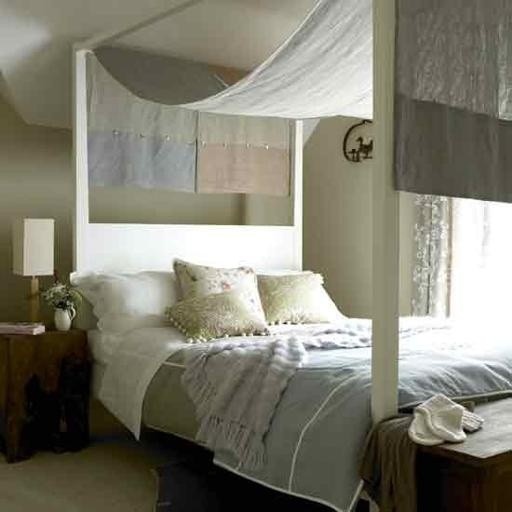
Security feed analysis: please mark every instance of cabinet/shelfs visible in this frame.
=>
[415,396,512,512]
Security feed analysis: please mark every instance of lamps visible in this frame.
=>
[12,218,54,322]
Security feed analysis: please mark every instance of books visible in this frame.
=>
[1,321,48,336]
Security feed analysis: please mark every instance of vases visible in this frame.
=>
[51,308,76,332]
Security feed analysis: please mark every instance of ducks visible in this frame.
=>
[356,137,373,158]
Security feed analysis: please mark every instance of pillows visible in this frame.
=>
[71,261,351,346]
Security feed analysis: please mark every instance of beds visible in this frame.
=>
[72,0,512,512]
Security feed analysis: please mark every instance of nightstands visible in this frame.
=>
[0,327,88,464]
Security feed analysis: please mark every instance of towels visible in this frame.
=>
[360,400,474,512]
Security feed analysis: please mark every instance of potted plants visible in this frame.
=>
[41,279,86,305]
